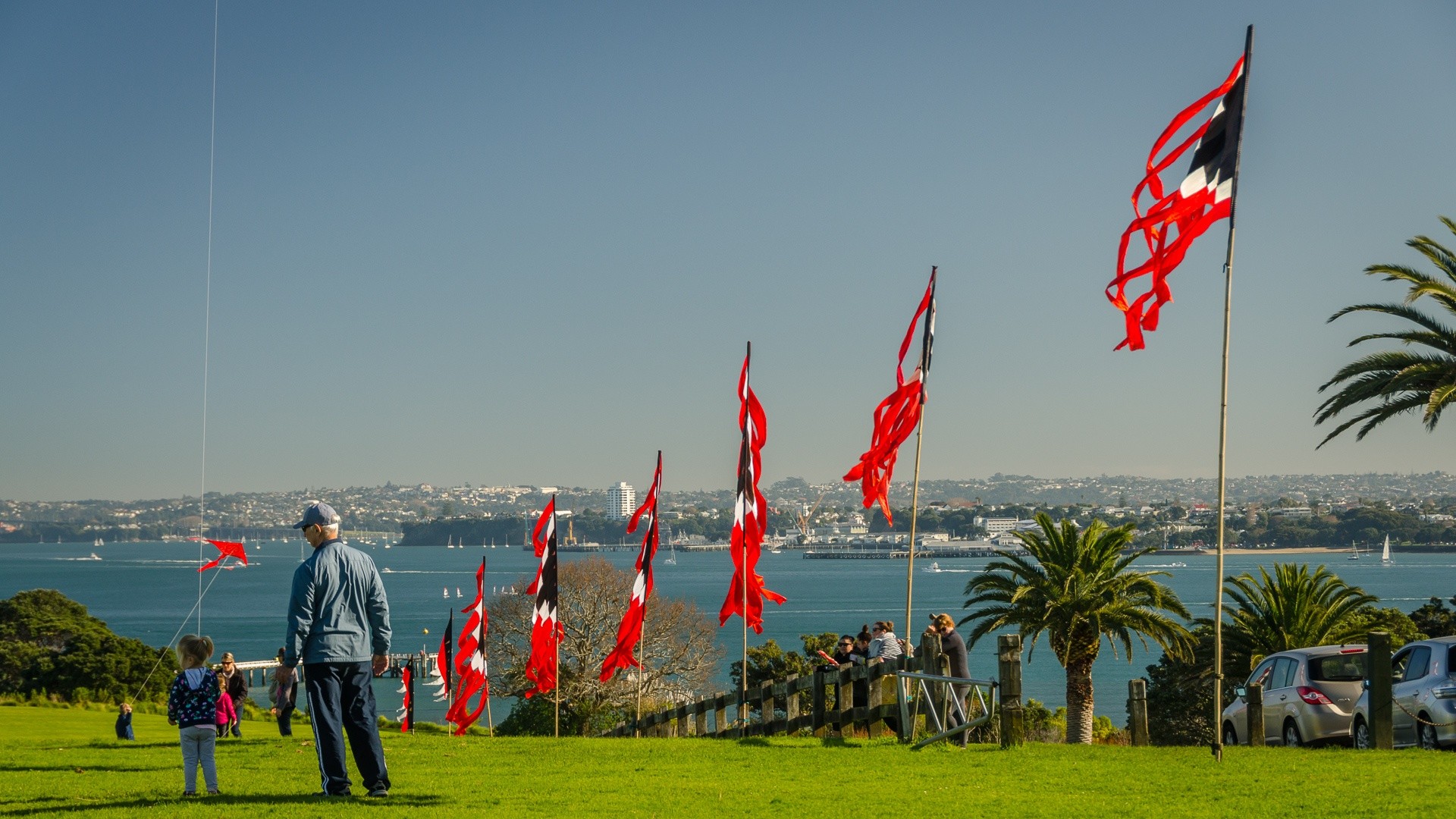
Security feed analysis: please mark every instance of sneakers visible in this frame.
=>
[209,790,221,795]
[183,791,195,796]
[369,780,388,797]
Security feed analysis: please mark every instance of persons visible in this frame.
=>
[168,634,221,796]
[274,502,393,796]
[214,653,248,738]
[269,647,299,737]
[828,614,971,745]
[115,703,135,740]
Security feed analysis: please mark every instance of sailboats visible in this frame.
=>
[443,586,450,599]
[1347,540,1359,560]
[37,525,398,562]
[493,585,519,596]
[448,535,510,549]
[455,586,463,598]
[1377,534,1395,565]
[666,536,676,565]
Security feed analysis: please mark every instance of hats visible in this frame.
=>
[929,614,940,620]
[293,503,338,529]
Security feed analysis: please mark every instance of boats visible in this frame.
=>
[924,562,940,572]
[382,568,390,571]
[1172,561,1186,568]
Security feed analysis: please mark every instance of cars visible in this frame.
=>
[1351,635,1456,753]
[1220,644,1407,751]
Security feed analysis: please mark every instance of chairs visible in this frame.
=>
[1339,664,1359,676]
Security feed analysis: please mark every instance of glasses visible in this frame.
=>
[302,525,311,532]
[221,662,232,665]
[838,642,851,647]
[873,627,882,631]
